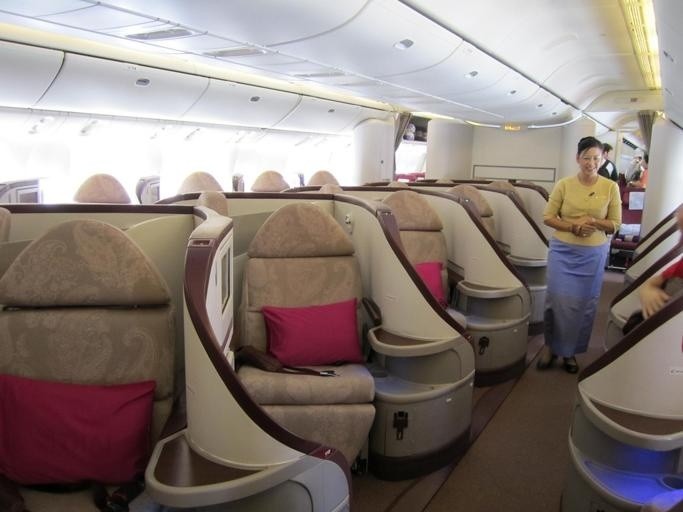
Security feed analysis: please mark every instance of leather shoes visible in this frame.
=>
[537,354,578,373]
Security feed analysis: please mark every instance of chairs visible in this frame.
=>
[446,183,497,243]
[0,217,174,512]
[230,201,377,471]
[379,189,468,333]
[387,180,407,187]
[307,169,339,186]
[489,180,524,208]
[73,173,131,206]
[178,171,222,190]
[197,190,228,217]
[604,187,646,270]
[251,170,293,192]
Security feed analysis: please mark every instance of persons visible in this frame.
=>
[639,206,683,321]
[536,137,623,373]
[627,154,648,189]
[596,144,619,184]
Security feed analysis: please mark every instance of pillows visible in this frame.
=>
[1,373,157,488]
[261,297,365,368]
[413,261,448,311]
